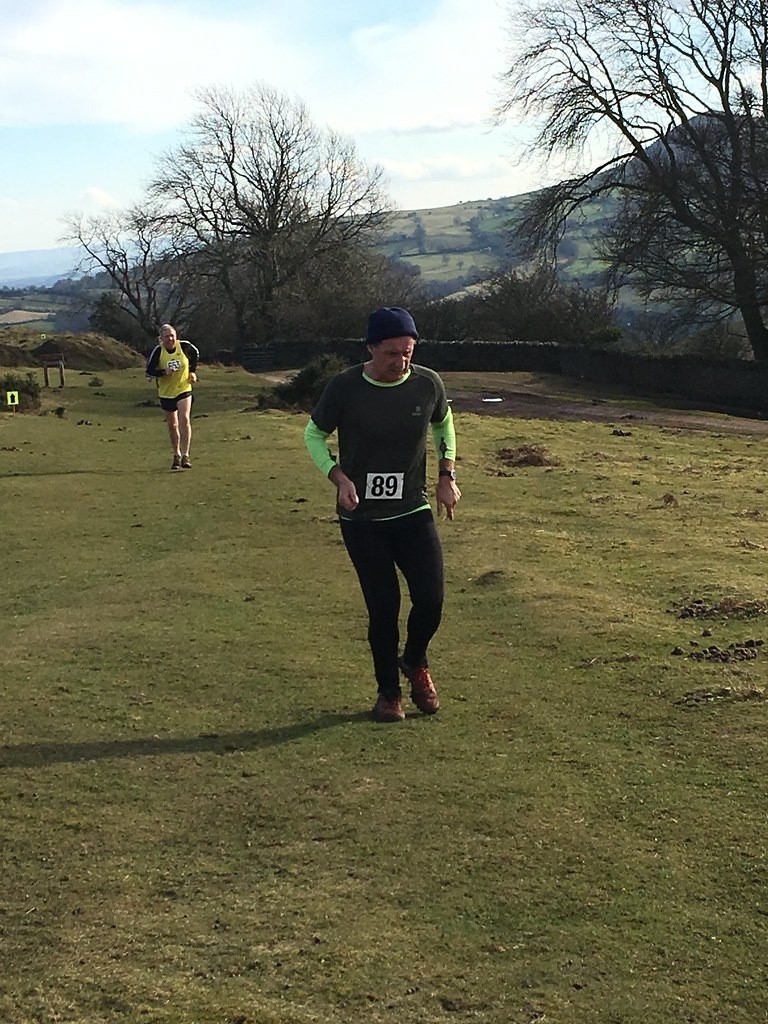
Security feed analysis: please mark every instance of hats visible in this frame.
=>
[366,307,419,343]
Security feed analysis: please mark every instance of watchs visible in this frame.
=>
[439,470,456,480]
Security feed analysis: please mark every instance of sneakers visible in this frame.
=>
[172,455,182,469]
[397,655,439,714]
[181,455,192,468]
[371,692,405,722]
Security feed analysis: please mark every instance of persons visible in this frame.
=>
[305,307,462,723]
[145,324,199,470]
